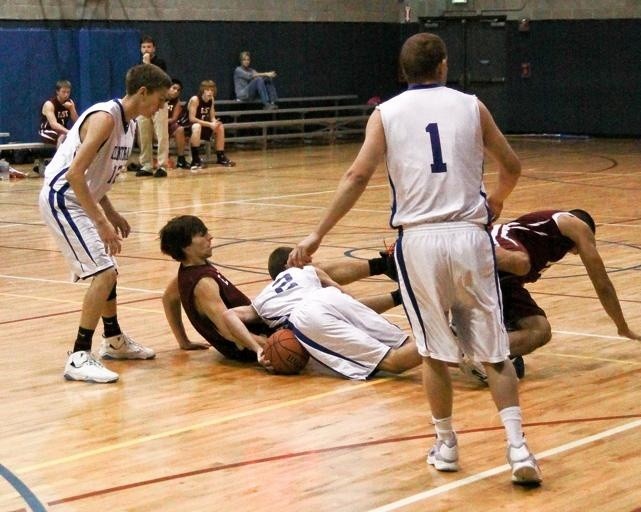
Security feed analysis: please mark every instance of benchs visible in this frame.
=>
[0,95,379,163]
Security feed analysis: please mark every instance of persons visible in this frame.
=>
[461,208,639,388]
[232,48,279,109]
[224,247,461,380]
[135,37,170,177]
[284,33,542,486]
[39,63,173,385]
[179,79,236,170]
[126,79,190,171]
[159,215,401,362]
[37,80,79,151]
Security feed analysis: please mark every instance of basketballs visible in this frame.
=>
[263,329,309,375]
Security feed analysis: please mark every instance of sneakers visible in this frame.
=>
[426,440,459,472]
[126,162,140,172]
[271,103,278,109]
[176,160,190,168]
[262,103,274,109]
[217,156,235,167]
[63,350,119,383]
[154,169,166,177]
[190,159,199,169]
[135,170,152,176]
[505,443,543,485]
[100,334,155,360]
